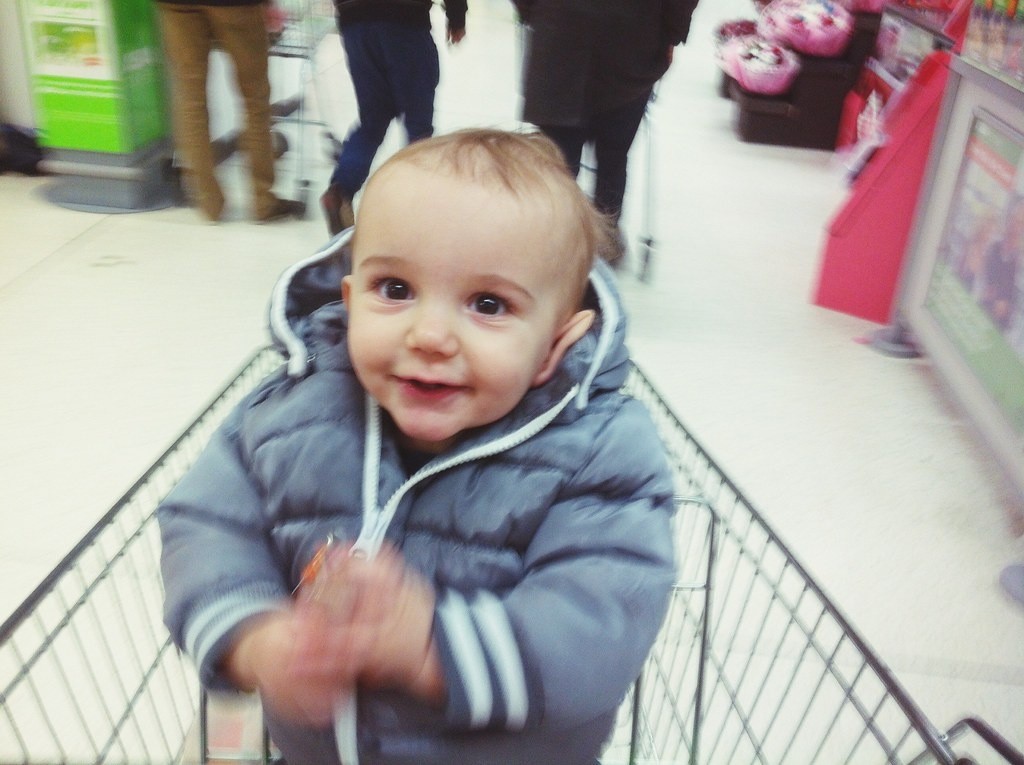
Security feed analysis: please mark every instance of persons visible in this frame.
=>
[950,188,1024,336]
[159,126,676,765]
[156,0,304,224]
[320,0,469,238]
[514,0,697,263]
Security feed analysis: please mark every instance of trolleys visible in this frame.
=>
[0,341,1024,765]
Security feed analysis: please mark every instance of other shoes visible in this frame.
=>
[248,198,306,225]
[186,195,224,225]
[604,225,627,265]
[319,174,355,237]
